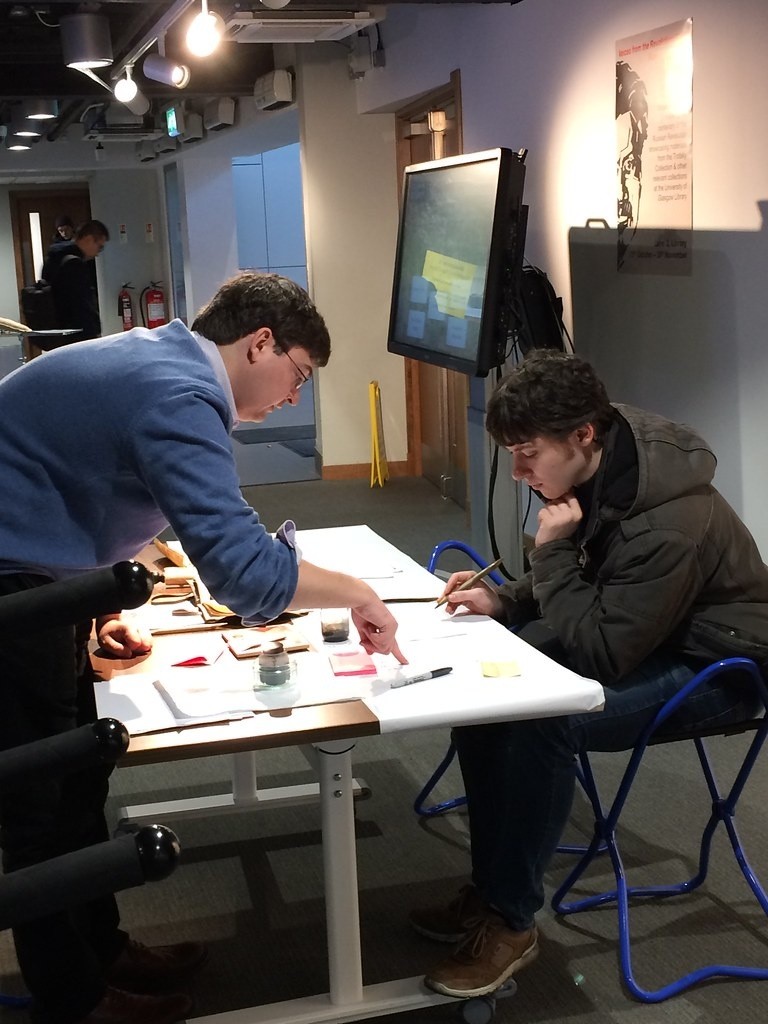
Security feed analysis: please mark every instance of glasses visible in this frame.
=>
[92,234,104,252]
[275,333,310,389]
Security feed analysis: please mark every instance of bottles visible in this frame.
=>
[258,643,290,685]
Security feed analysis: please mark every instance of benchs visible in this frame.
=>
[413,539,767,1002]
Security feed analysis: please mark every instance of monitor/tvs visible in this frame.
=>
[387,146,529,377]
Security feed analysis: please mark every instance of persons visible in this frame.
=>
[0,267,409,1024]
[35,216,75,289]
[41,219,110,346]
[410,346,768,995]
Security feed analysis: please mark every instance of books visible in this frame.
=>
[221,626,310,659]
[185,579,226,623]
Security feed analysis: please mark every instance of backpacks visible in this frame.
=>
[21,255,81,351]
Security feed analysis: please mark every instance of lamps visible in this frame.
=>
[7,100,59,152]
[94,140,106,164]
[58,13,114,70]
[110,0,225,116]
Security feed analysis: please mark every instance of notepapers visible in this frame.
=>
[328,651,378,676]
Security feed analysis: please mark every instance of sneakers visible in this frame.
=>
[423,913,539,998]
[79,984,194,1024]
[96,935,197,992]
[407,884,501,942]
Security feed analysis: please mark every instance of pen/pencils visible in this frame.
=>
[434,557,504,610]
[390,667,453,690]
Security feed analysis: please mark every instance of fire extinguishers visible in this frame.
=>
[139,279,165,329]
[118,281,135,332]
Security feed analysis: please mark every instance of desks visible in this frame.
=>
[89,525,606,1024]
